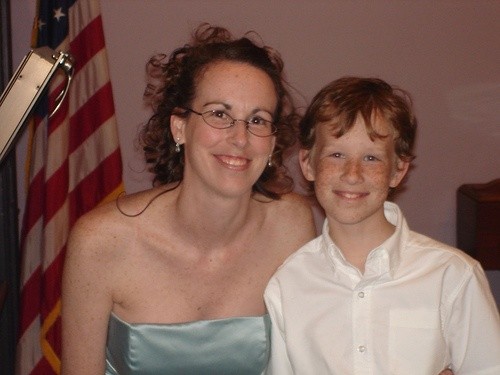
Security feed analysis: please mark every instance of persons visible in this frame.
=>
[262,78,500,375]
[61,22,457,375]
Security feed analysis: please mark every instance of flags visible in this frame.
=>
[17,0,125,375]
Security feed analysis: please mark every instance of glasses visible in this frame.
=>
[187,108,279,137]
